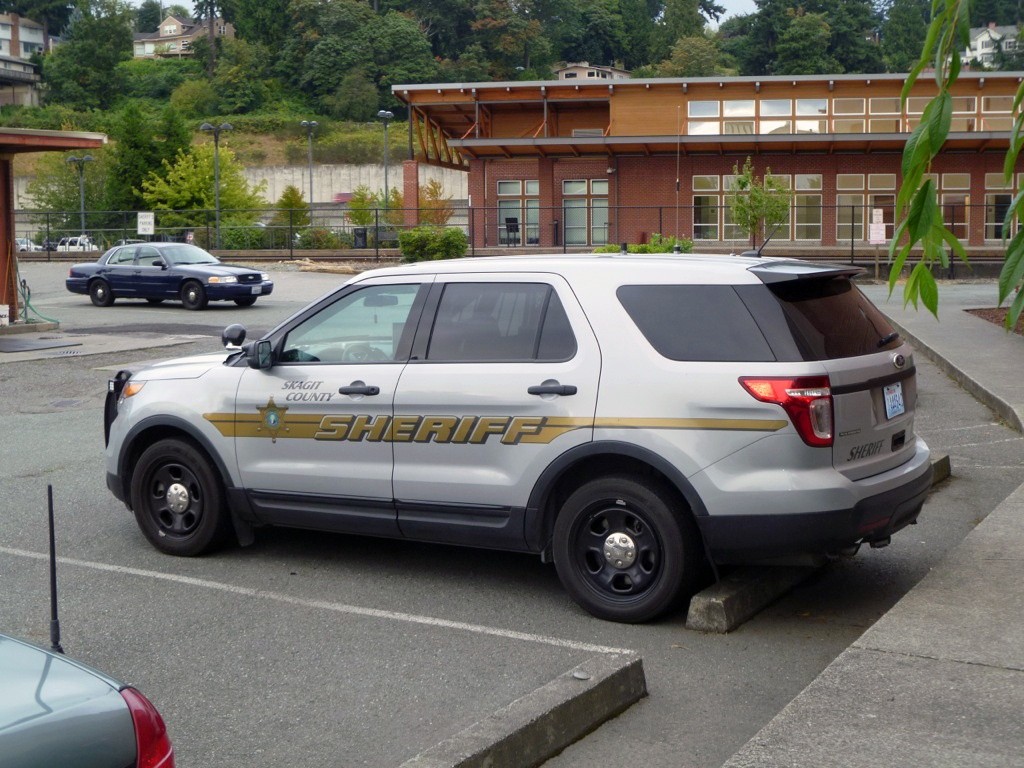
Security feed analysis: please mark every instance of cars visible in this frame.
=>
[60,240,274,311]
[17,238,42,259]
[58,237,98,255]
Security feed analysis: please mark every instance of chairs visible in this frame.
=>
[428,316,502,359]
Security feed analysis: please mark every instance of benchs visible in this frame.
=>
[371,232,398,248]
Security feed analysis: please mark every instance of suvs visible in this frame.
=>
[102,251,936,624]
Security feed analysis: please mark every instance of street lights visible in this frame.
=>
[295,118,323,253]
[370,106,399,251]
[202,120,235,248]
[66,153,95,239]
